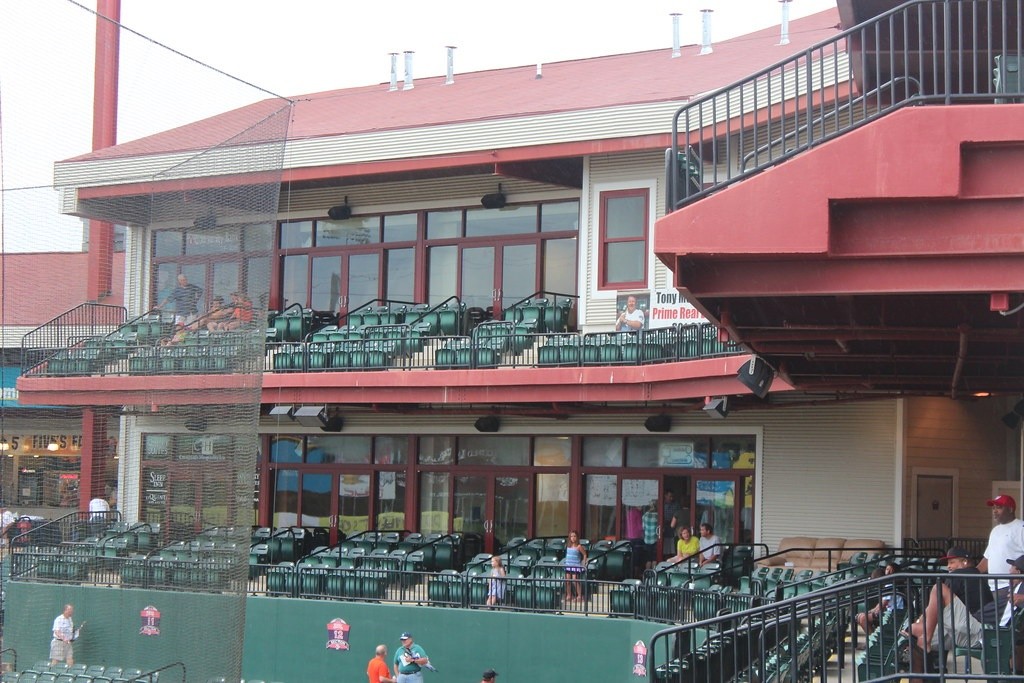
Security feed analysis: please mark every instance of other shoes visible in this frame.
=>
[561,596,584,602]
[857,640,867,650]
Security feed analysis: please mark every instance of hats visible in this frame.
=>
[987,495,1016,510]
[483,669,498,678]
[400,632,411,640]
[1006,555,1024,572]
[940,547,968,559]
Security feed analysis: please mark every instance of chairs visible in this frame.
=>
[45,296,742,373]
[0,522,1024,683]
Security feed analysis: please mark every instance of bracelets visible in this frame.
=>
[413,659,415,663]
[624,319,628,324]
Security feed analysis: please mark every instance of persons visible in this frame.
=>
[561,529,588,603]
[394,632,429,683]
[90,495,111,541]
[616,296,645,332]
[46,603,76,669]
[151,274,254,352]
[855,495,1024,683]
[367,645,397,683]
[482,669,498,683]
[626,489,753,570]
[486,556,507,609]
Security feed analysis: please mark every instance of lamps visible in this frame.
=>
[736,353,777,400]
[269,403,295,422]
[702,395,729,419]
[292,403,329,428]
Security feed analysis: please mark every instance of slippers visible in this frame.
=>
[900,631,925,655]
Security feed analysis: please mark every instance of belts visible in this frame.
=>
[57,639,74,642]
[401,670,420,675]
